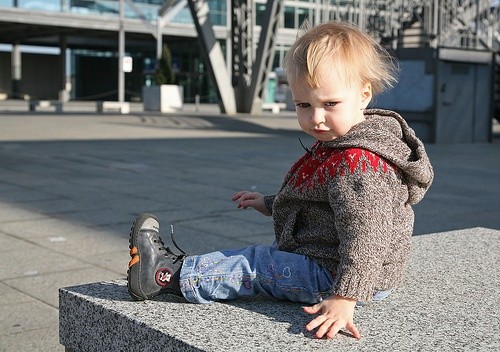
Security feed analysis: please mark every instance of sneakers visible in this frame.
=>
[126,212,189,301]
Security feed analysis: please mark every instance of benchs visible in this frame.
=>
[28,99,65,116]
[93,100,130,117]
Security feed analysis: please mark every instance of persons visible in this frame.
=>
[127,17,434,339]
